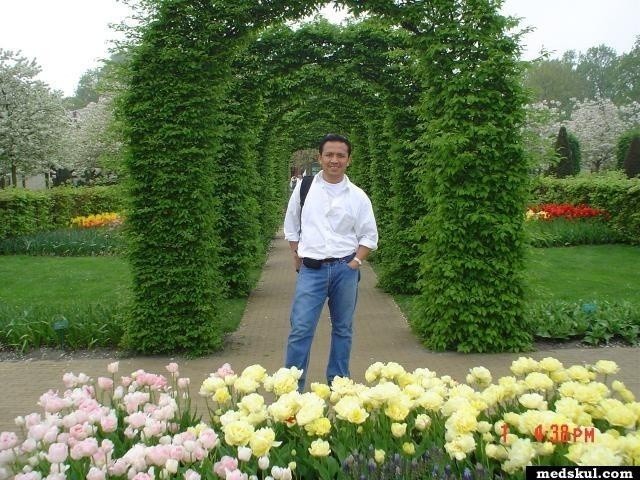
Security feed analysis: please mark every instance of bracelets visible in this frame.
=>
[353,257,362,266]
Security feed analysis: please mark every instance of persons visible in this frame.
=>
[284,135,379,395]
